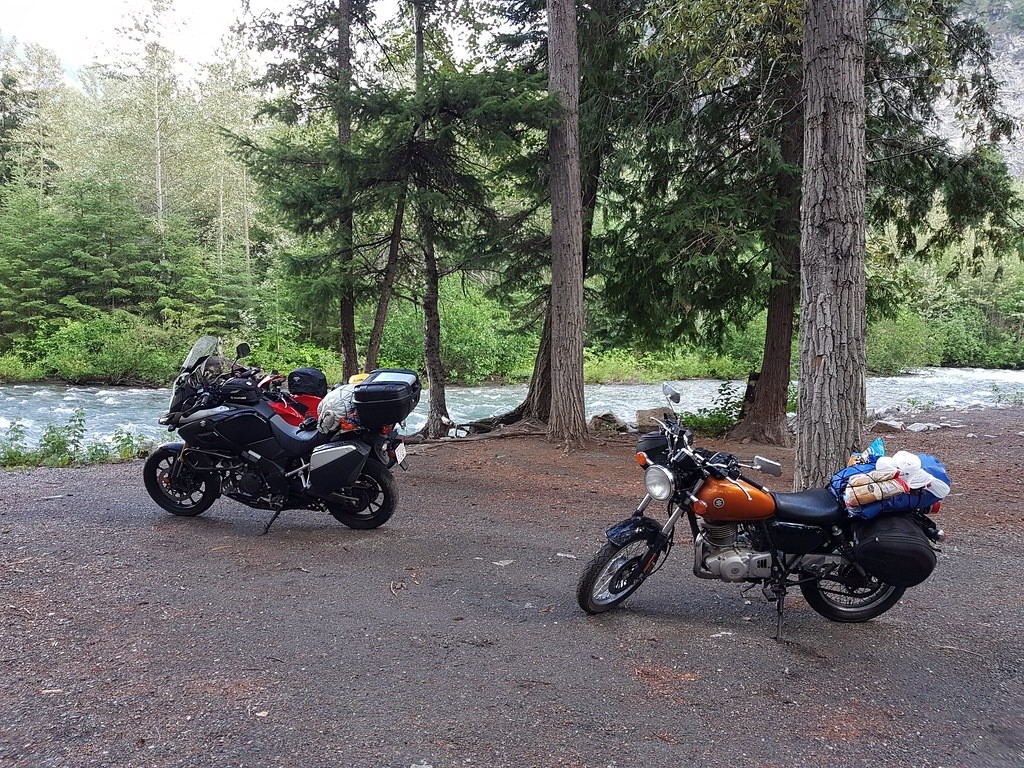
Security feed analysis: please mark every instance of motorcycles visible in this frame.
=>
[143,336,421,539]
[573,382,947,644]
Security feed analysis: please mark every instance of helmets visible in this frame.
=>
[637,432,672,470]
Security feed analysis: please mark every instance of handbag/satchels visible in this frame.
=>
[288,367,327,397]
[851,511,943,587]
[220,378,265,403]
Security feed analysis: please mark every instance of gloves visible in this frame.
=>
[701,451,741,478]
